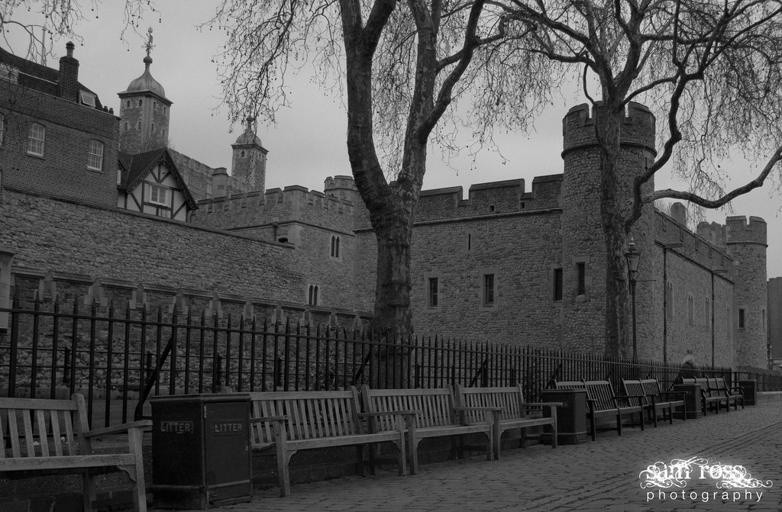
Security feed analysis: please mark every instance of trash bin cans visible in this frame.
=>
[675,384,703,420]
[739,381,756,405]
[149,394,252,512]
[542,389,586,444]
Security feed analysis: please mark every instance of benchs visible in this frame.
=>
[1,393,152,512]
[556,371,745,442]
[224,378,567,498]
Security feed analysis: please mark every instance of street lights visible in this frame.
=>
[624,237,641,372]
